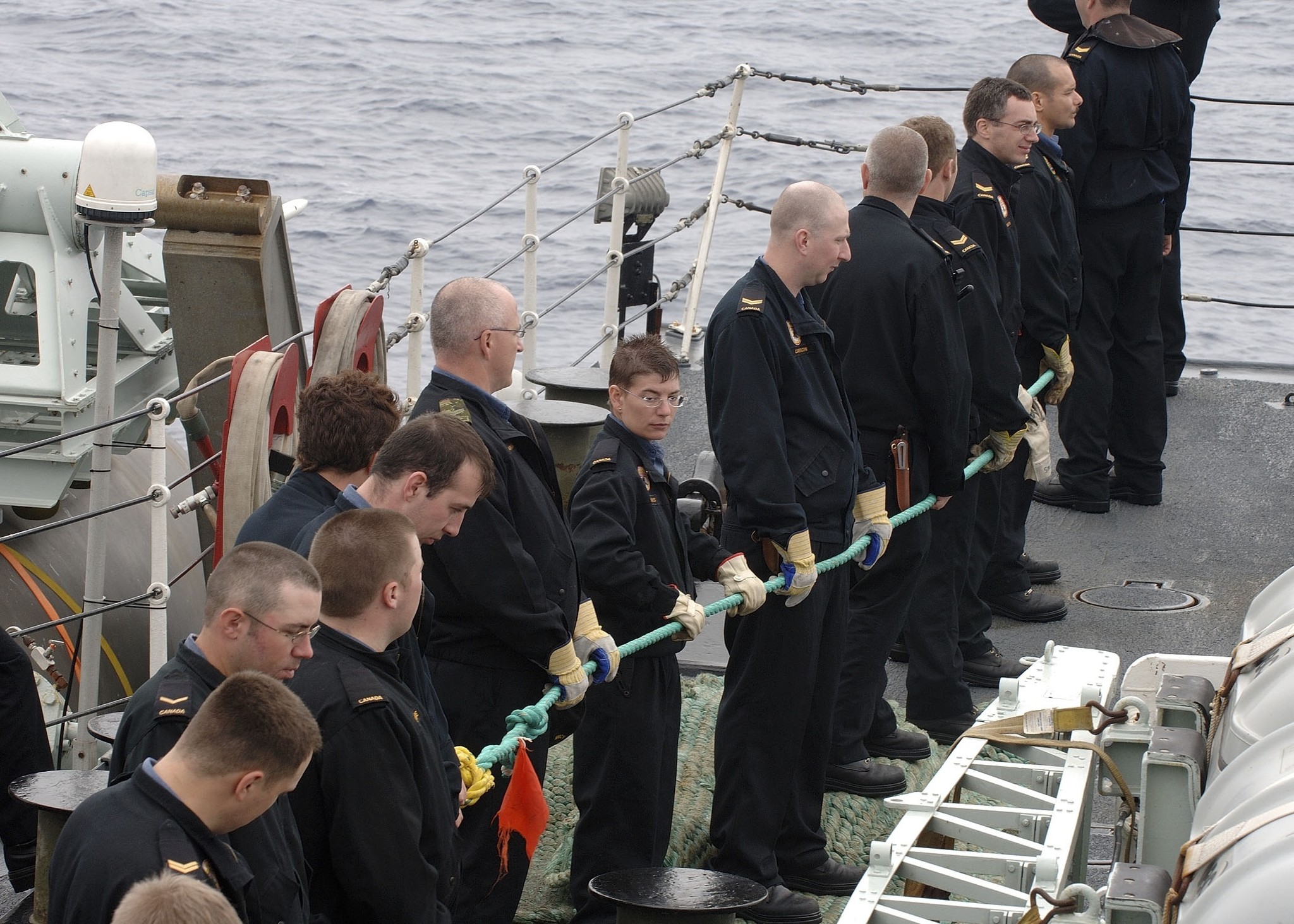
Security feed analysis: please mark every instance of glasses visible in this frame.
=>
[618,386,688,408]
[243,610,322,645]
[473,326,526,340]
[984,118,1044,135]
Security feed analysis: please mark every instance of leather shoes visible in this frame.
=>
[736,553,1069,923]
[1031,379,1179,514]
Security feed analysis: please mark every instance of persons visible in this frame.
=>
[706,178,894,923]
[809,3,1225,794]
[408,280,622,924]
[562,332,766,912]
[34,370,499,924]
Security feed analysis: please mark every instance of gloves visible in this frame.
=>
[662,583,704,642]
[541,631,589,711]
[849,481,892,571]
[768,526,818,608]
[571,596,620,687]
[714,551,766,618]
[966,422,1028,474]
[1015,384,1052,482]
[1038,333,1075,405]
